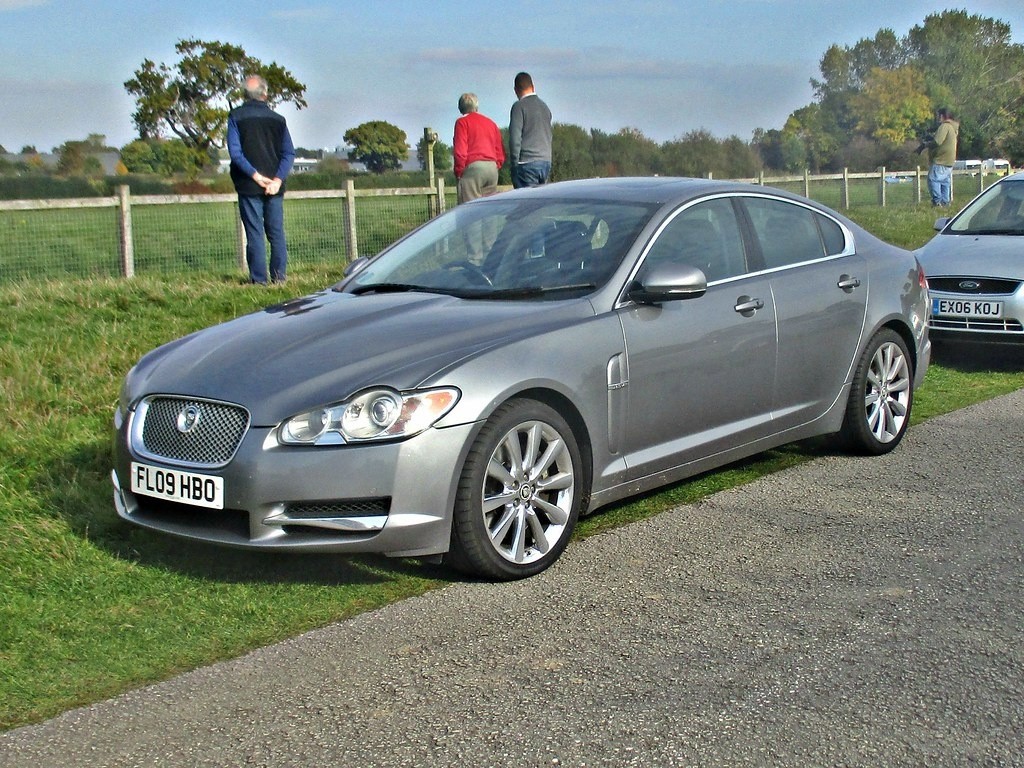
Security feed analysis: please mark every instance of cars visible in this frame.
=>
[110,172,933,581]
[912,170,1024,352]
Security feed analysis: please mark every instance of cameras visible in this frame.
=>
[916,135,933,154]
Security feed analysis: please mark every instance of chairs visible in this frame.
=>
[507,220,592,286]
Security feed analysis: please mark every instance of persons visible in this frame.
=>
[921,108,962,207]
[509,70,554,260]
[452,93,506,269]
[227,74,296,283]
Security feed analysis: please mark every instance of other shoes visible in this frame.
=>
[241,279,284,286]
